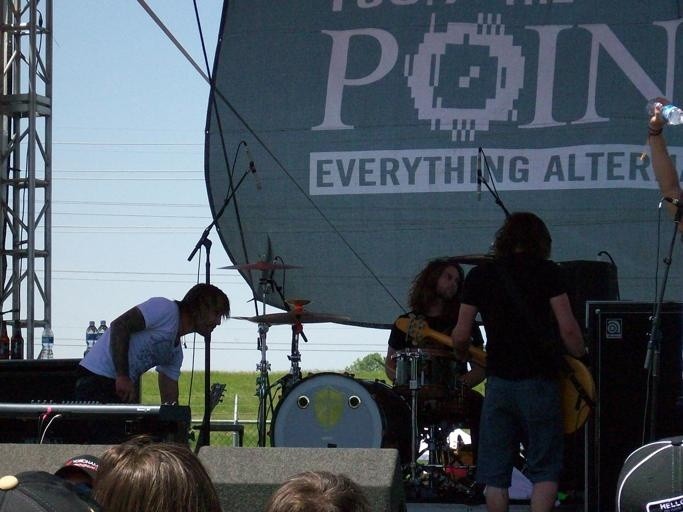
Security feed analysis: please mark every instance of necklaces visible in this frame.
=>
[179,315,188,350]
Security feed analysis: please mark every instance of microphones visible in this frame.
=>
[243,146,264,192]
[264,259,279,295]
[476,146,501,202]
[659,195,683,207]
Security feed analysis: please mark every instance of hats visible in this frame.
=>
[0,470,98,511]
[53,454,99,478]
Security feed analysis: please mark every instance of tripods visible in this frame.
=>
[399,381,474,504]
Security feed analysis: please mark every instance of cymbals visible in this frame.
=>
[218,264,303,270]
[249,313,351,323]
[452,254,489,264]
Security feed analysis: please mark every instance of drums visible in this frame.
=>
[270,372,418,464]
[394,349,465,401]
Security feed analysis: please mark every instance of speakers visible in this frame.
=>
[586,300,682,512]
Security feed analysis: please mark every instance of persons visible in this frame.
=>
[0,456,103,511]
[75,282,230,405]
[92,436,219,512]
[270,469,370,512]
[644,97,682,235]
[382,257,486,466]
[450,210,589,510]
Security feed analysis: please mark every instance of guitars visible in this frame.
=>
[396,315,594,435]
[194,383,227,453]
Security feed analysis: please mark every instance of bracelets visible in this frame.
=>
[646,124,663,137]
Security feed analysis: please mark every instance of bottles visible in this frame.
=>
[99,320,108,335]
[0,321,9,360]
[647,98,683,124]
[86,321,98,352]
[11,320,23,359]
[41,325,54,359]
